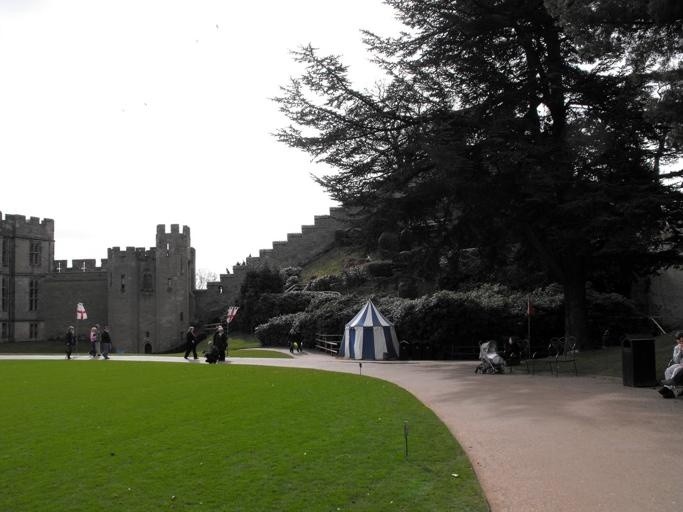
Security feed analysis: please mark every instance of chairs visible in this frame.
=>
[473,334,577,378]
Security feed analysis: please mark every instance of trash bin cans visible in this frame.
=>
[399,340,433,360]
[621,334,657,387]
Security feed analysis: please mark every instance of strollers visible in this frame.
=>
[473,341,506,375]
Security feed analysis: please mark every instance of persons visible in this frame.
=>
[100,326,113,359]
[503,335,521,364]
[88,327,96,357]
[184,326,199,359]
[287,335,302,354]
[660,370,682,386]
[92,323,102,357]
[62,325,75,359]
[659,332,682,399]
[213,325,227,362]
[487,344,505,365]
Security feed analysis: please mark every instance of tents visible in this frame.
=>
[337,298,400,360]
